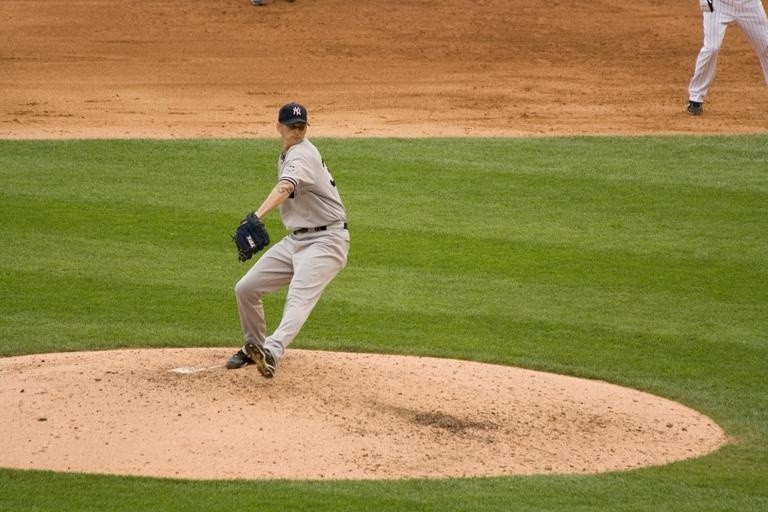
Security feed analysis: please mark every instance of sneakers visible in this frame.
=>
[687,99,704,115]
[226,349,254,368]
[245,342,276,376]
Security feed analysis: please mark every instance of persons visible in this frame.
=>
[684,0,767,117]
[224,101,349,378]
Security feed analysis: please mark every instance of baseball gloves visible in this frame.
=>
[236,213,270,262]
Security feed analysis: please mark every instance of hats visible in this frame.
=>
[279,103,310,126]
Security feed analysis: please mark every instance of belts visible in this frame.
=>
[299,223,348,233]
